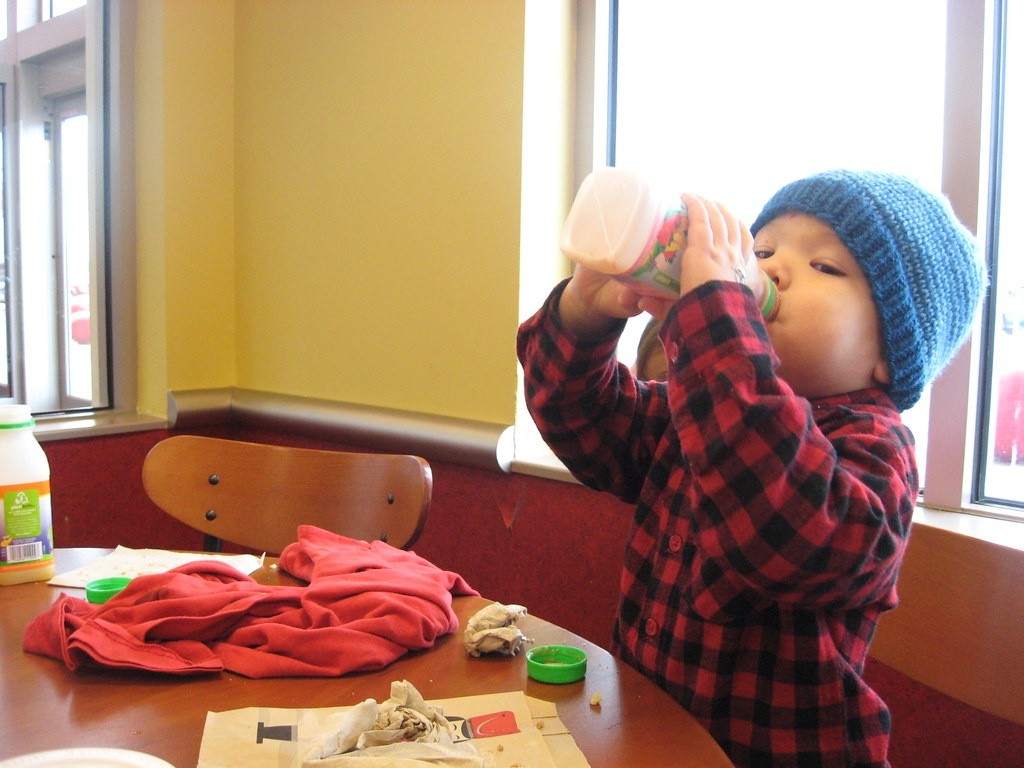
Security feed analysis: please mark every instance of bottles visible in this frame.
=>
[0,404,55,586]
[562,165,780,324]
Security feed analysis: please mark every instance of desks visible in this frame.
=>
[0,548,737,768]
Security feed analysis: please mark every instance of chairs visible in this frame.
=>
[142,435,432,554]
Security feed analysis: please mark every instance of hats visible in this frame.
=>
[750,169,987,413]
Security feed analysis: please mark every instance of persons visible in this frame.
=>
[636,317,668,382]
[518,169,988,767]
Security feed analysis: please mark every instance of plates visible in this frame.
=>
[0,747,175,768]
